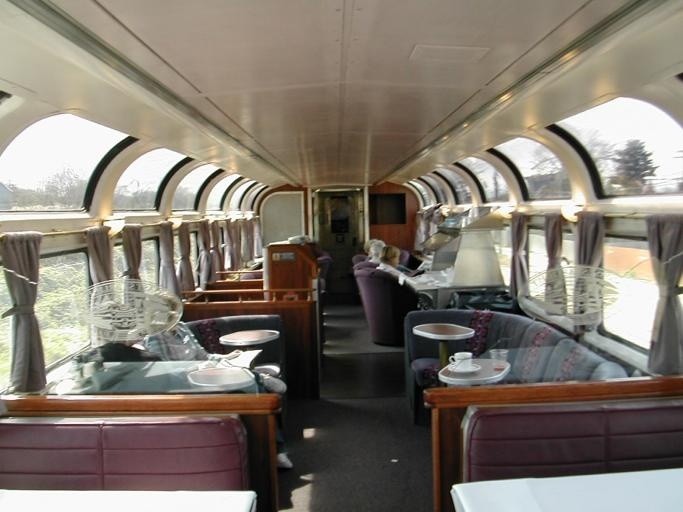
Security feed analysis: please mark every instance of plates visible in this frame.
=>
[448,363,480,372]
[188,367,254,386]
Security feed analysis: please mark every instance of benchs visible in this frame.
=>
[0,267,321,512]
[424,376,683,512]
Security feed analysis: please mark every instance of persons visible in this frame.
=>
[287,234,409,283]
[145,294,294,469]
[87,304,158,361]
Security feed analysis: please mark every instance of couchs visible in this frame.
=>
[404,310,629,421]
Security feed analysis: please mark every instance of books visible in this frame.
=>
[220,348,263,370]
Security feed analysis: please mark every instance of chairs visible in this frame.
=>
[351,249,412,344]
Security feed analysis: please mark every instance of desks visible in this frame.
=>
[438,358,511,385]
[452,468,683,512]
[413,324,476,387]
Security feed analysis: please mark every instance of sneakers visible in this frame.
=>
[258,374,286,394]
[276,453,292,469]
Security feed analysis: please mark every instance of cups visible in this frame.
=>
[449,352,472,368]
[489,348,508,371]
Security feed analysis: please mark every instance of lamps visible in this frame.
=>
[100,215,215,239]
[499,203,585,223]
